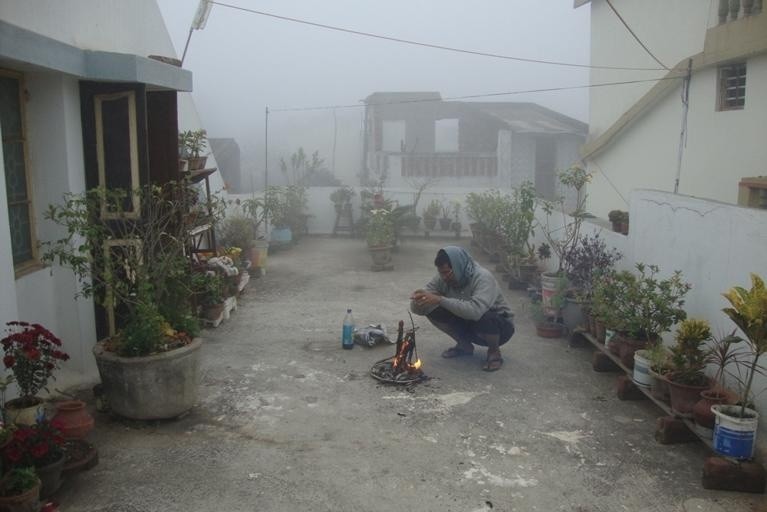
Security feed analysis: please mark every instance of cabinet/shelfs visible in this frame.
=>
[182,168,217,270]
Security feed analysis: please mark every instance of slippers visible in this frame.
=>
[441,348,473,358]
[483,358,503,372]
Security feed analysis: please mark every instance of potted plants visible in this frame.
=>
[37,179,211,420]
[218,183,315,268]
[0,467,41,512]
[331,185,461,263]
[178,131,228,225]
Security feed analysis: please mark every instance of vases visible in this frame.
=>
[54,401,95,441]
[208,303,224,321]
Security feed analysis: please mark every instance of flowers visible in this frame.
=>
[0,321,57,460]
[207,247,242,303]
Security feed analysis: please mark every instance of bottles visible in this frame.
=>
[343,309,354,349]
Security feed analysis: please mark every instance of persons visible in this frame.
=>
[410,246,515,373]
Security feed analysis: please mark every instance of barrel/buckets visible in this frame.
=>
[541,272,564,312]
[632,350,658,388]
[712,405,758,460]
